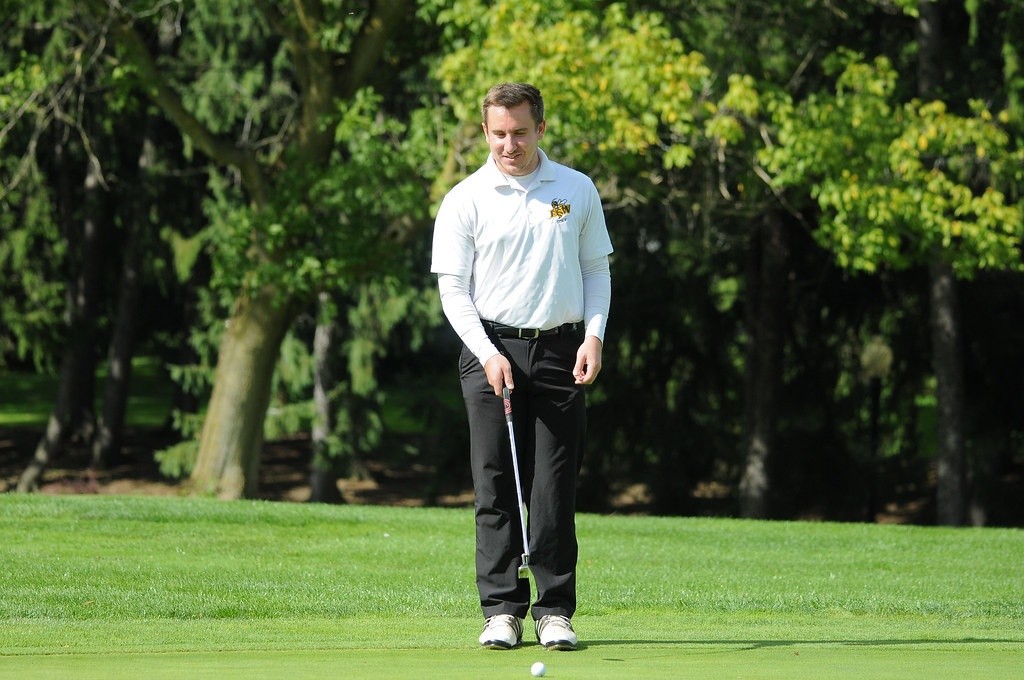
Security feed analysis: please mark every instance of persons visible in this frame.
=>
[431,83,614,652]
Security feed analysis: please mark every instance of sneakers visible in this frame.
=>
[534,613,578,650]
[478,614,524,650]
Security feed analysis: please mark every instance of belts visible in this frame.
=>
[481,316,584,340]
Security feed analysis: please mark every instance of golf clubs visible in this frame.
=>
[501,385,531,580]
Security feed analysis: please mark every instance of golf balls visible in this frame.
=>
[531,662,546,676]
